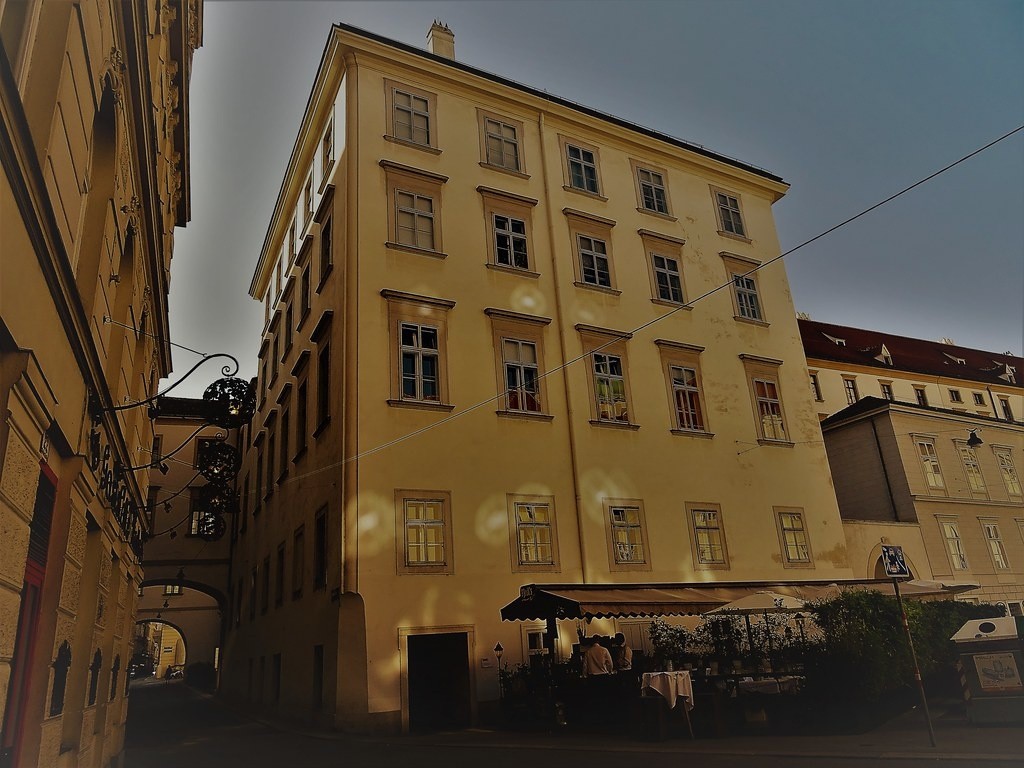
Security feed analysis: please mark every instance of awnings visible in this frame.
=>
[498,581,954,679]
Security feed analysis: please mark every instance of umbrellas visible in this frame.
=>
[905,580,980,603]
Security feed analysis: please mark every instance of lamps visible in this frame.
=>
[134,443,238,515]
[119,392,242,485]
[138,482,228,543]
[98,315,258,431]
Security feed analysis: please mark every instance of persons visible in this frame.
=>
[582,633,634,720]
[164,663,173,685]
